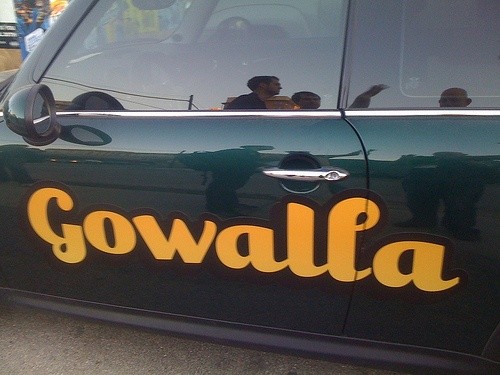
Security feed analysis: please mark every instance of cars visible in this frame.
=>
[0,0,500,375]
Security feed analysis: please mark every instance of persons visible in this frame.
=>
[225,75,284,110]
[350,86,387,109]
[437,87,473,107]
[292,90,322,110]
[377,117,488,241]
